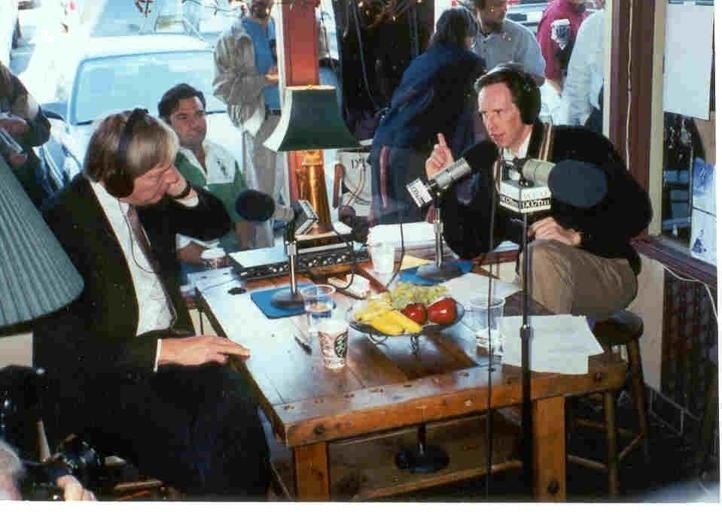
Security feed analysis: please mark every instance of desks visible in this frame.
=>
[196,253,628,499]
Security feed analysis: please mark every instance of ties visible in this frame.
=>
[127,205,191,338]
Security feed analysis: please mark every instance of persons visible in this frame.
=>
[1,62,58,210]
[157,82,257,286]
[468,0,547,88]
[366,8,486,223]
[32,108,272,497]
[210,1,291,249]
[0,442,98,500]
[534,1,588,94]
[564,2,606,133]
[425,62,652,317]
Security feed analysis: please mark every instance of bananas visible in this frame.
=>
[356,299,423,337]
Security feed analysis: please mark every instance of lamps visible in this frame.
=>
[260,82,355,244]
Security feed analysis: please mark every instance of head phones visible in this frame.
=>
[478,67,541,125]
[104,107,148,198]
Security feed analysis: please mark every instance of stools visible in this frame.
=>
[563,313,657,497]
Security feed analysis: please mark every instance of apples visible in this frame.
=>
[427,298,457,325]
[400,304,427,326]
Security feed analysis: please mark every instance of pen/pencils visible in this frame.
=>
[294,336,312,355]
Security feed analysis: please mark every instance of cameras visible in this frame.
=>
[17,438,105,501]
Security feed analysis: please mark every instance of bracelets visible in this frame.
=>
[173,181,191,199]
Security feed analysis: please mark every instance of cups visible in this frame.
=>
[297,281,336,327]
[314,318,349,369]
[469,293,505,352]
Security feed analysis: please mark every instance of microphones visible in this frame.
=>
[406,140,498,206]
[500,159,608,212]
[236,189,319,237]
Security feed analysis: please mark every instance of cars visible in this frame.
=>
[506,0,548,33]
[15,26,244,206]
